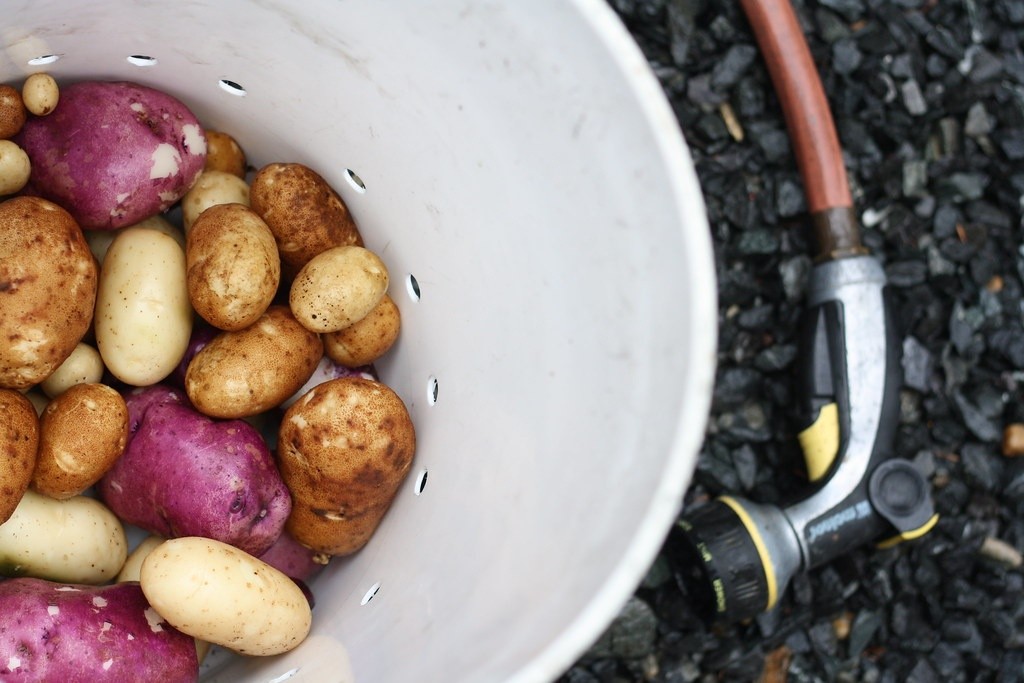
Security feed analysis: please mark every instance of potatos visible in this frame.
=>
[0,74,418,683]
